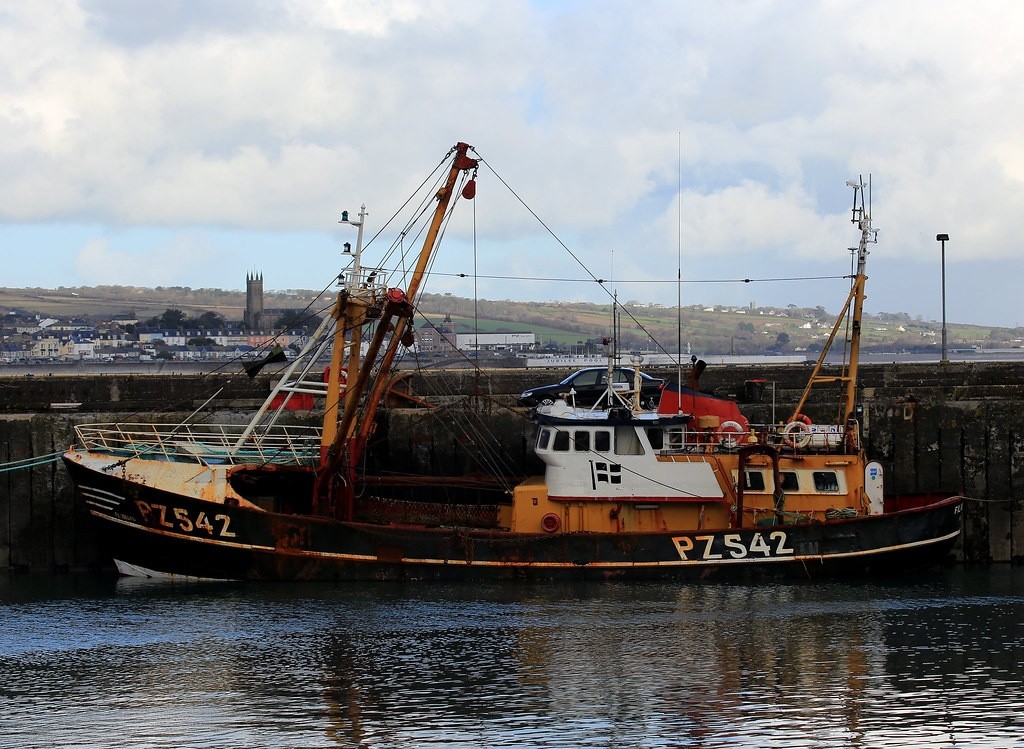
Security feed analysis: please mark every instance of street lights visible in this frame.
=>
[937,234,950,356]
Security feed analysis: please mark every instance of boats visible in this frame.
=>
[59,144,971,590]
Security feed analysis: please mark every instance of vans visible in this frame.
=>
[139,355,152,361]
[516,355,528,359]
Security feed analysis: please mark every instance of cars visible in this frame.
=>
[468,352,480,356]
[517,365,666,407]
[1,357,138,363]
[322,352,448,358]
[155,356,261,361]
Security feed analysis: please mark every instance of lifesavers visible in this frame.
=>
[787,414,812,436]
[782,421,811,448]
[717,420,744,449]
